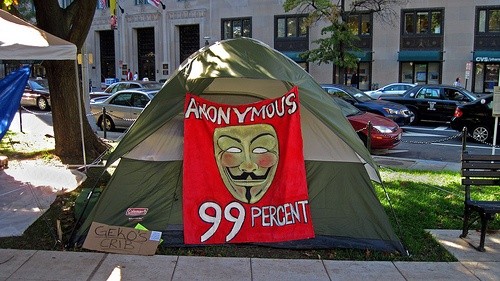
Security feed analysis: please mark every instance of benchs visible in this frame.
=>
[459,150,500,251]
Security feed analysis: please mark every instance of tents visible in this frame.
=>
[64,37,412,258]
[0,8,87,171]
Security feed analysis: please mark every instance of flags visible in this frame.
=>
[148,0,160,8]
[109,0,118,30]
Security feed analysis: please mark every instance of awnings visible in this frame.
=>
[398,49,443,61]
[346,49,372,62]
[473,51,500,63]
[279,51,305,61]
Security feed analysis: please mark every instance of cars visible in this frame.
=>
[20,77,51,111]
[450,93,500,144]
[331,95,402,154]
[89,80,162,100]
[353,83,439,100]
[90,89,160,131]
[320,84,415,127]
[377,83,480,121]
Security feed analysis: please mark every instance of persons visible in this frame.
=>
[143,74,150,81]
[134,71,139,80]
[454,77,462,86]
[126,68,132,80]
[351,73,357,89]
